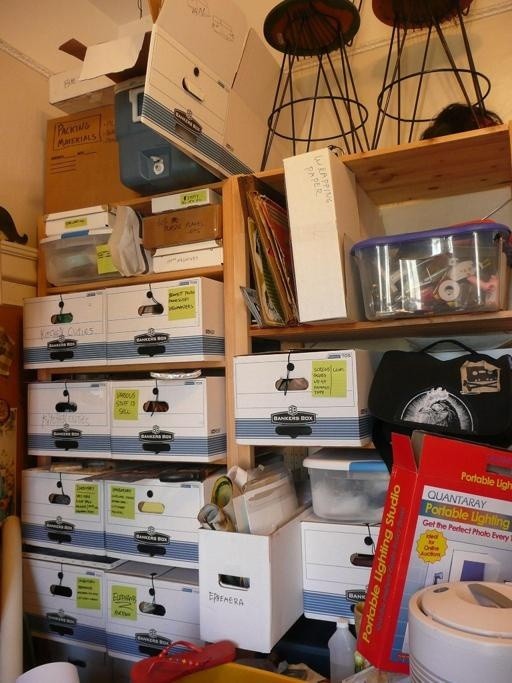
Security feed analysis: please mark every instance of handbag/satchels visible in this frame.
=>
[130,639,237,683]
[365,339,512,477]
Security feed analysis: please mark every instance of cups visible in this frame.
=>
[353,601,365,640]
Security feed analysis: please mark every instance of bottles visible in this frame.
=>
[327,618,357,682]
